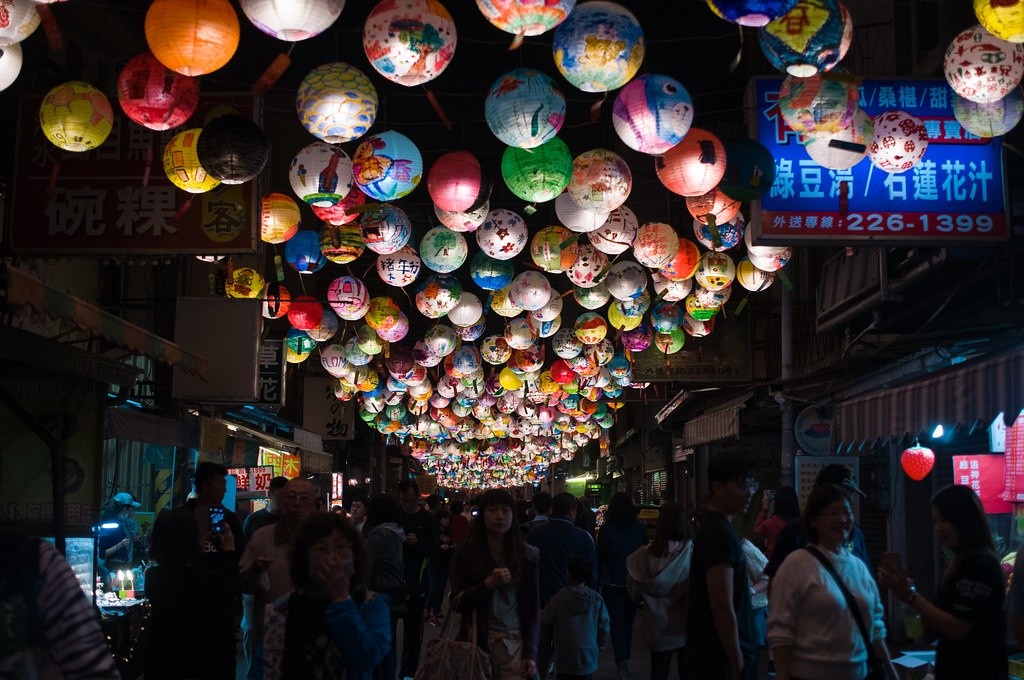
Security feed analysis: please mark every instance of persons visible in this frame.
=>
[0,539,123,680]
[210,513,224,525]
[143,462,245,680]
[237,463,897,680]
[100,493,141,571]
[879,484,1009,680]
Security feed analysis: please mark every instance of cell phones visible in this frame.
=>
[209,504,224,534]
[882,552,901,571]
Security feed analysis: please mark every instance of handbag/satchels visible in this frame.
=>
[413,591,493,680]
[866,661,890,680]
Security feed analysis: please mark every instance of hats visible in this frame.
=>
[115,492,140,507]
[816,463,868,501]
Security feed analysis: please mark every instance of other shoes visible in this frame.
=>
[616,661,630,680]
[429,617,440,627]
[423,608,430,621]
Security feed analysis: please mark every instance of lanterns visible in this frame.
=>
[0,0,63,90]
[115,0,926,491]
[943,0,1024,138]
[39,82,113,151]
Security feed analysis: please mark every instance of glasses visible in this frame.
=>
[818,506,855,519]
[720,479,760,496]
[399,496,419,505]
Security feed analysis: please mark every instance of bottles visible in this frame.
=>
[898,577,923,638]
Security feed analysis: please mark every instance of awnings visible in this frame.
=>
[0,262,208,390]
[833,347,1024,451]
[200,407,333,477]
[683,395,756,451]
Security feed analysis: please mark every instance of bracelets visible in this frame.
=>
[899,585,918,602]
